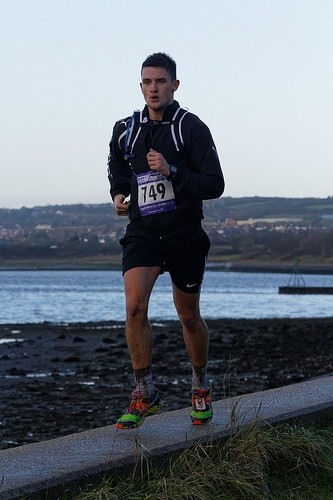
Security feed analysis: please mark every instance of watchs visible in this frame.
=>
[167,164,178,177]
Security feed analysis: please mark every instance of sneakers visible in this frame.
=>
[189,386,214,426]
[116,385,162,431]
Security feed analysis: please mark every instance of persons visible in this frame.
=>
[105,54,226,431]
